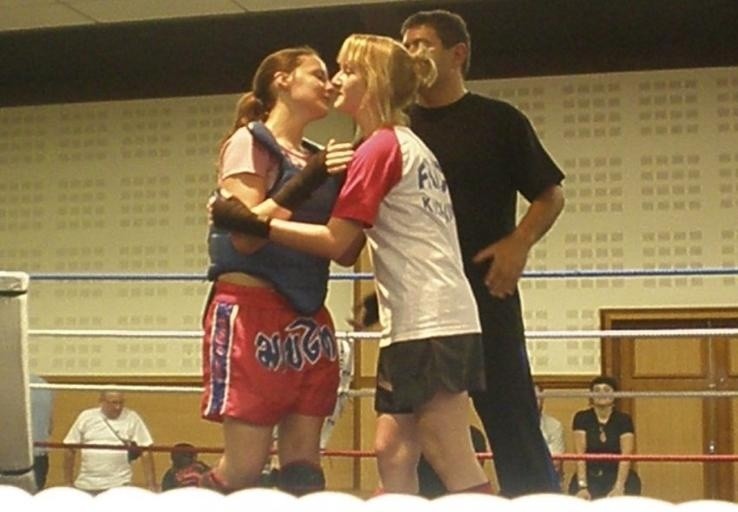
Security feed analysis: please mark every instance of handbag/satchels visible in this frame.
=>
[127,439,143,463]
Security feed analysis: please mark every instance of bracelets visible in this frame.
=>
[575,478,588,491]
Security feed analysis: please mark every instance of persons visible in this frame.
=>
[24,370,54,492]
[194,42,366,494]
[530,380,566,484]
[60,378,163,493]
[161,441,210,489]
[342,7,569,497]
[205,33,495,499]
[564,376,642,497]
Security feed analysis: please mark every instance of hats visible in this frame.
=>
[591,377,618,393]
